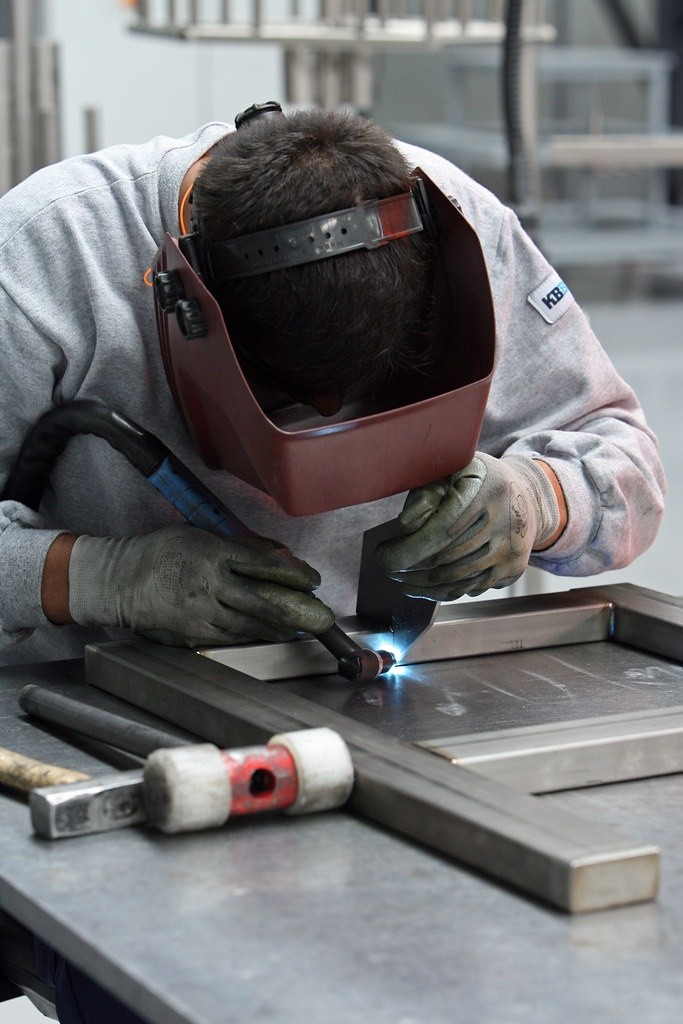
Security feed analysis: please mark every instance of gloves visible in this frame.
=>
[67,520,335,649]
[371,449,562,603]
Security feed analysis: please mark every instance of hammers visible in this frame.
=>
[17,682,355,834]
[0,746,145,839]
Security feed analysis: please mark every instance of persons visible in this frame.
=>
[0,101,668,668]
